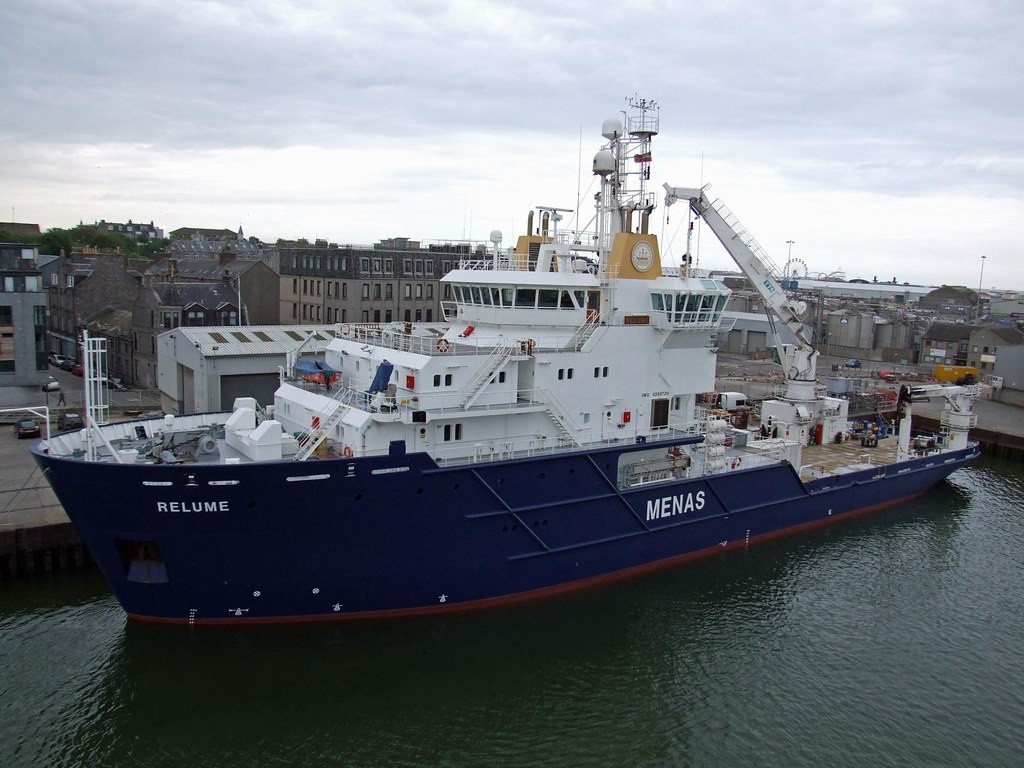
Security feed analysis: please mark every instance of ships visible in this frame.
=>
[0,85,983,628]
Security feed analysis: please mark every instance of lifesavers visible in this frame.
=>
[731,456,742,469]
[343,446,353,457]
[436,338,450,352]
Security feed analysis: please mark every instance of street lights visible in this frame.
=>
[785,240,796,291]
[974,255,987,326]
[298,274,306,325]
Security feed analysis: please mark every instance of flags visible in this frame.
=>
[634,152,652,163]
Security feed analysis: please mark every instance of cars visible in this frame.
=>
[48,349,132,393]
[56,412,85,432]
[42,376,61,392]
[845,359,862,368]
[15,418,42,439]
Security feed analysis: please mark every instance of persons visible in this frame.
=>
[867,438,876,448]
[57,390,68,406]
